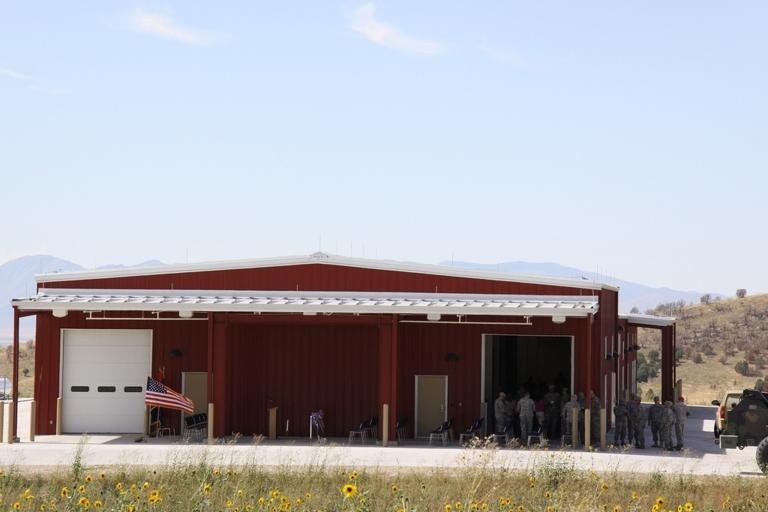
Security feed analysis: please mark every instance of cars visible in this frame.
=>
[0,377,13,400]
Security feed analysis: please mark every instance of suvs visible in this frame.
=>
[709,390,768,441]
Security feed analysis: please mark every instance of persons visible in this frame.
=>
[492,381,691,451]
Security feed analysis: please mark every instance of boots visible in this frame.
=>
[614,440,645,448]
[652,440,683,451]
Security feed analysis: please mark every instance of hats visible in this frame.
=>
[664,401,673,407]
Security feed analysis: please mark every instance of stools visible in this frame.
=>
[154,424,176,443]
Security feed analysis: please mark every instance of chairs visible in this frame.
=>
[347,414,552,450]
[182,413,206,444]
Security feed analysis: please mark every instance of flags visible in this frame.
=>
[142,376,195,415]
